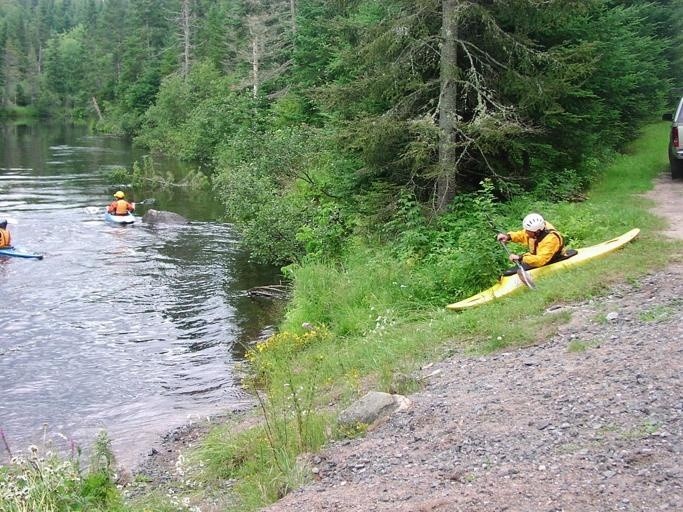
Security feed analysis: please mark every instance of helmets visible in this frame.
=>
[523,213,546,236]
[113,191,125,199]
[0,219,8,227]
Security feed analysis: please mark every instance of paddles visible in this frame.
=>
[484,213,536,291]
[84,198,156,213]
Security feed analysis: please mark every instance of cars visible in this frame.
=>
[660,96,682,182]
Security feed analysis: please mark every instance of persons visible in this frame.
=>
[105,191,135,217]
[0,220,10,249]
[495,211,567,280]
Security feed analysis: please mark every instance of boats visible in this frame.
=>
[104,207,137,225]
[439,225,642,313]
[0,245,46,262]
